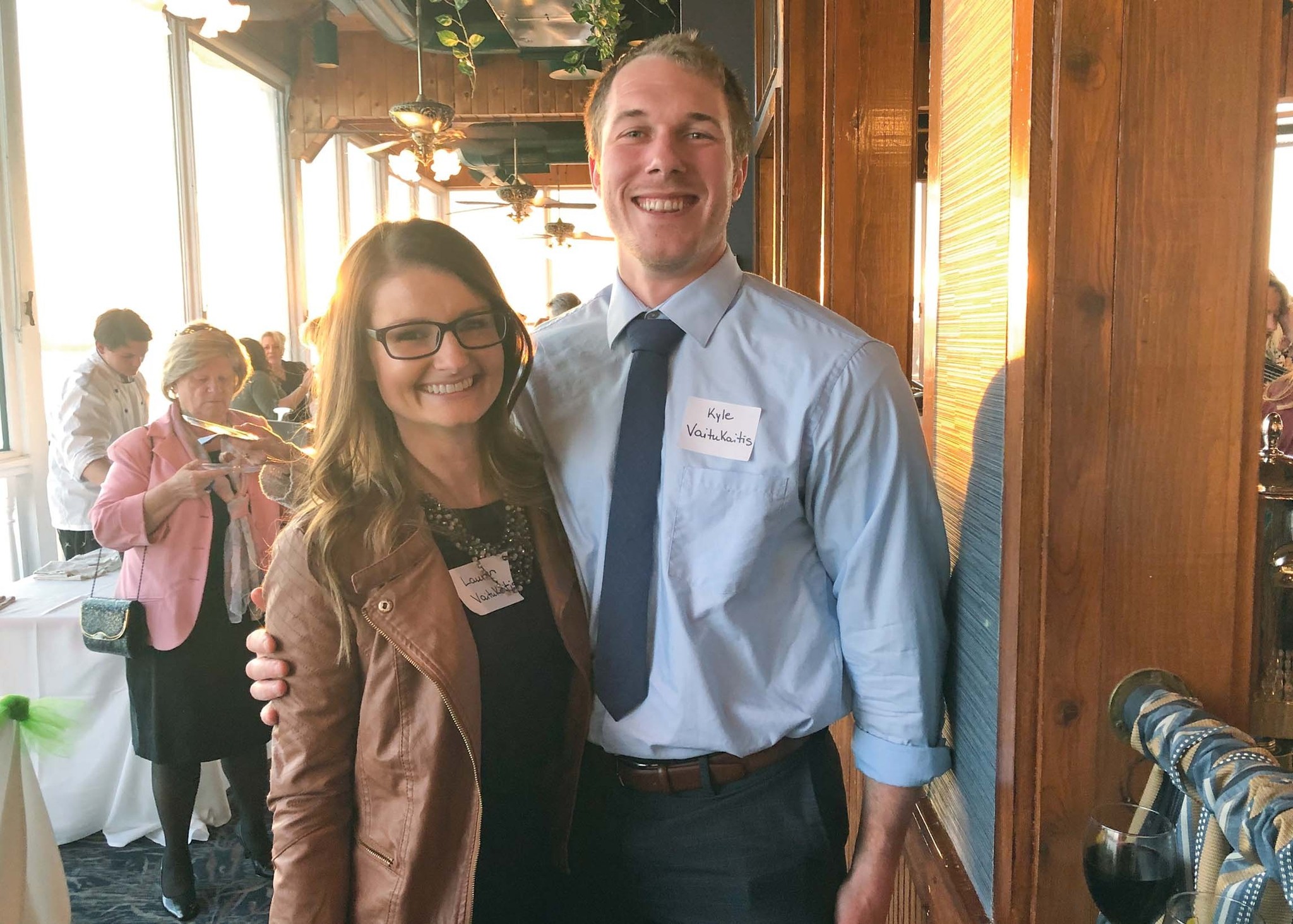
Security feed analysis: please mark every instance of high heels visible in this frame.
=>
[159,855,197,921]
[236,820,273,876]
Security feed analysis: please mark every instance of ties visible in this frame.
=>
[590,316,686,722]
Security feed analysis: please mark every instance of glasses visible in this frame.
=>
[362,309,512,360]
[179,324,220,335]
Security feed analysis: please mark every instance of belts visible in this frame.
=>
[586,729,818,794]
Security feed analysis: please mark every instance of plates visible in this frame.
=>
[201,462,262,474]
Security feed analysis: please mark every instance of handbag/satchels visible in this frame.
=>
[81,597,146,658]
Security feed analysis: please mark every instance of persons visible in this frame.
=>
[46,292,580,922]
[247,34,954,924]
[272,221,593,924]
[1265,268,1293,366]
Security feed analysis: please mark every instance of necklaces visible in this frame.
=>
[418,487,533,592]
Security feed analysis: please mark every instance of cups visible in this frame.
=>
[1161,891,1266,924]
[1082,802,1176,923]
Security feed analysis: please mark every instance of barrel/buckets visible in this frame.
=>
[1247,411,1293,759]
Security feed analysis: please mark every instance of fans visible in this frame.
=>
[304,0,545,164]
[522,165,615,248]
[447,122,596,216]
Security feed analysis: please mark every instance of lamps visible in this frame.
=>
[389,147,462,182]
[310,0,339,68]
[507,212,529,224]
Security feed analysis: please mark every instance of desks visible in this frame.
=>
[1,547,232,849]
